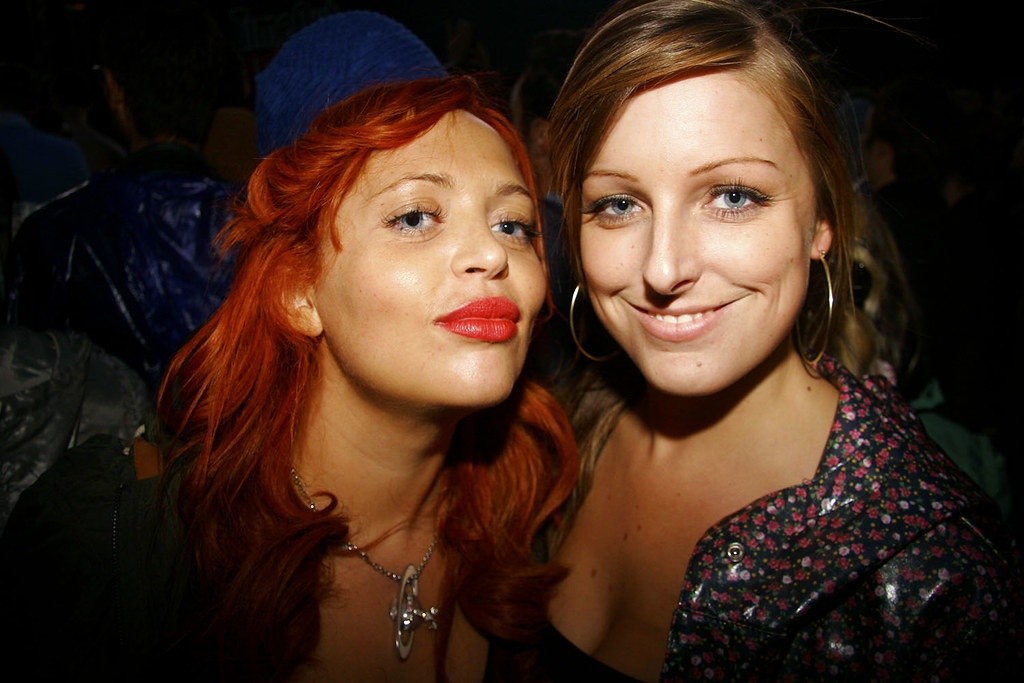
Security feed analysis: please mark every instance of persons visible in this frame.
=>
[506,28,591,304]
[487,0,1022,682]
[0,0,247,388]
[253,11,453,162]
[0,322,181,683]
[158,74,580,683]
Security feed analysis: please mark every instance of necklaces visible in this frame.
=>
[289,465,443,659]
